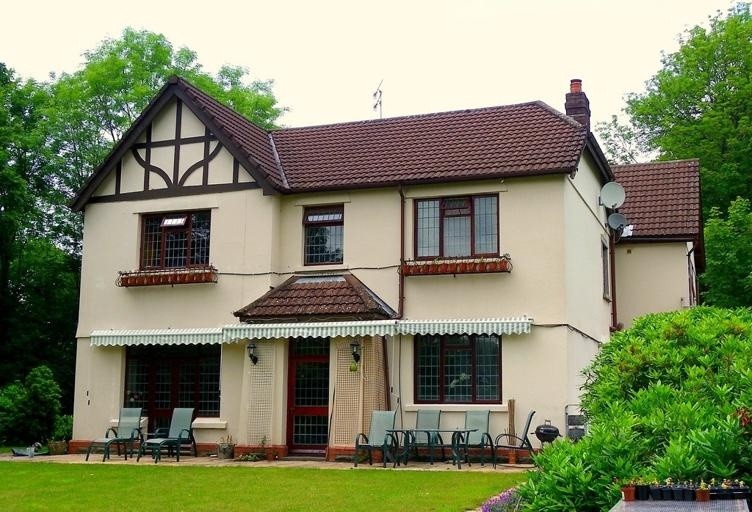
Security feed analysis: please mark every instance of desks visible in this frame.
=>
[608,497,749,512]
[385,427,480,469]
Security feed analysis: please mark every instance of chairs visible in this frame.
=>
[494,409,535,468]
[354,411,398,468]
[136,408,197,463]
[453,410,493,465]
[564,405,588,441]
[86,408,144,462]
[404,409,445,462]
[620,477,748,502]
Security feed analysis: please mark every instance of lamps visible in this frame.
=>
[247,343,257,365]
[350,340,360,361]
